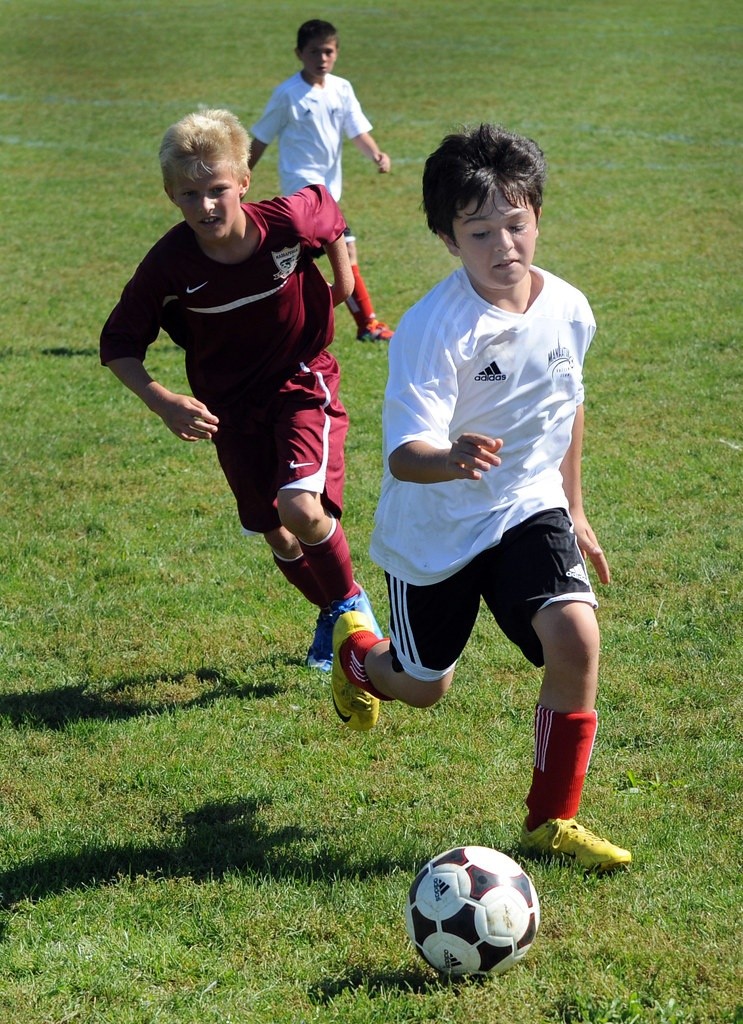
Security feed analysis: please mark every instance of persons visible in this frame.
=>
[329,120,637,876]
[95,105,384,683]
[245,17,394,342]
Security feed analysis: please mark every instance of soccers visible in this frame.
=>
[404,847,540,978]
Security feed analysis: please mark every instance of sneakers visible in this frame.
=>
[357,322,394,341]
[520,815,633,872]
[304,608,334,672]
[329,610,380,732]
[331,580,384,640]
